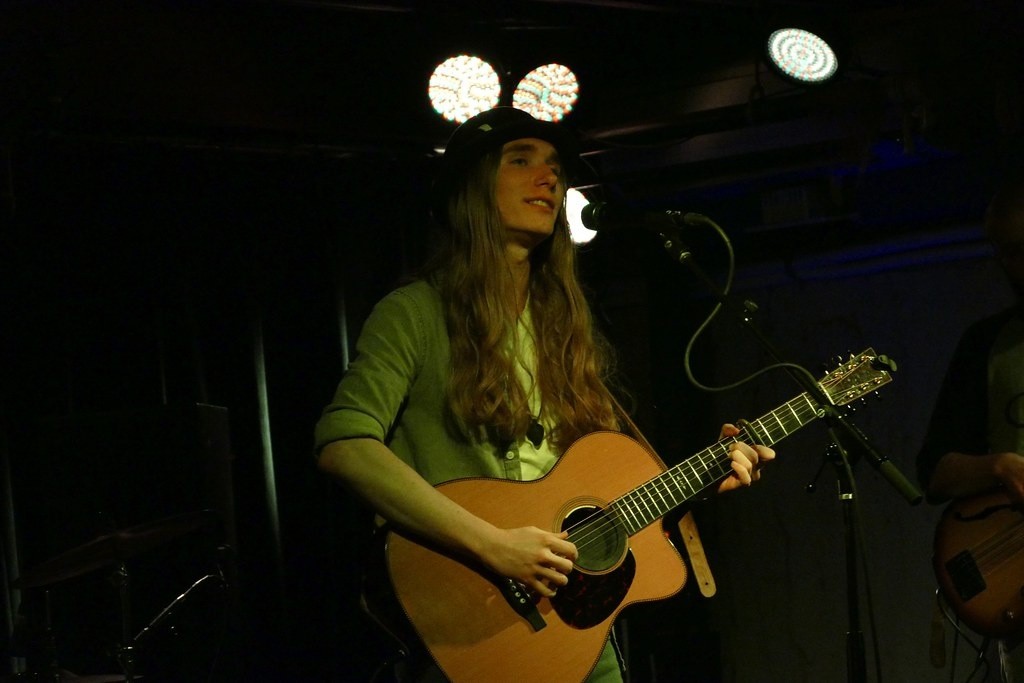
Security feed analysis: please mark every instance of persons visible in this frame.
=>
[915,155,1024,683]
[315,104,776,683]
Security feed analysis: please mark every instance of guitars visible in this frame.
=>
[366,346,902,681]
[930,480,1023,639]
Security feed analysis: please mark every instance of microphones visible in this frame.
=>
[581,201,713,232]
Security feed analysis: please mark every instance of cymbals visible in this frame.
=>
[22,504,224,589]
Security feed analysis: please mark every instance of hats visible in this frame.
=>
[428,106,572,226]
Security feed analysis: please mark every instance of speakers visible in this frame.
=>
[122,575,412,683]
